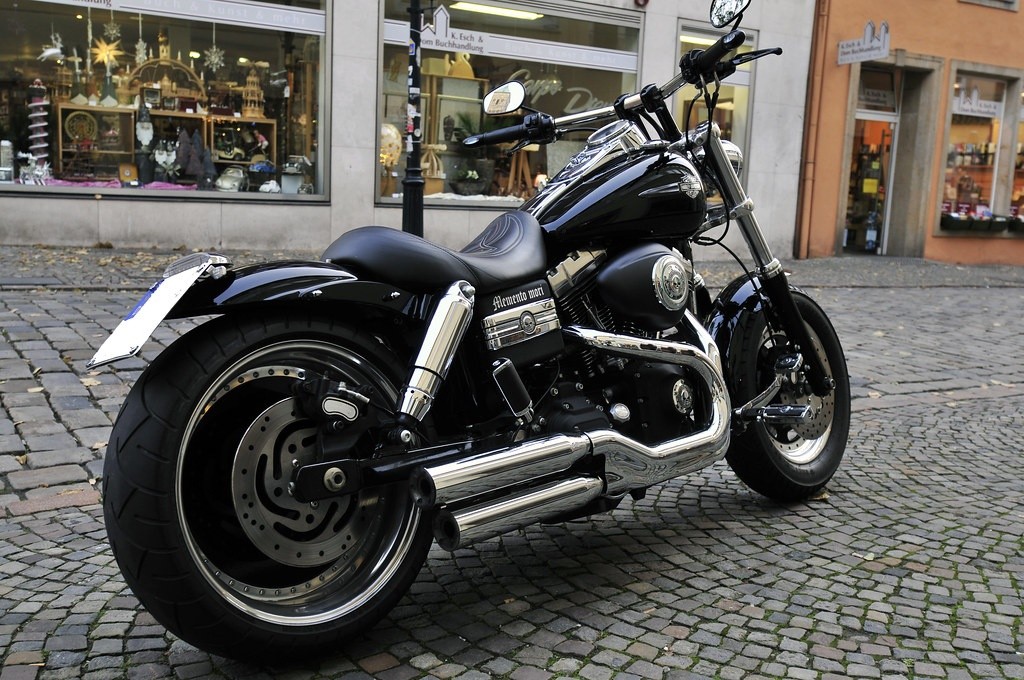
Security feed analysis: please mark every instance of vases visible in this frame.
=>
[450,181,487,195]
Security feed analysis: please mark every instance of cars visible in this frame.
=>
[260,179,281,193]
[214,164,249,192]
[250,160,275,173]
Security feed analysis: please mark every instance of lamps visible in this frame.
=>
[379,123,403,197]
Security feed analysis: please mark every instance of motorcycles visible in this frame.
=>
[85,0,852,664]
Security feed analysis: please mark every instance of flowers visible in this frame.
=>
[454,163,487,181]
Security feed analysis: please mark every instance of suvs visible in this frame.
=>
[282,155,306,172]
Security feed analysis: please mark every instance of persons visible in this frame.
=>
[253,130,268,154]
[136,111,180,183]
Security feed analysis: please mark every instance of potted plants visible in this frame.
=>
[456,112,516,195]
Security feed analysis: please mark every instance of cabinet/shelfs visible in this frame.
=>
[51,102,134,180]
[135,109,206,183]
[208,115,276,186]
